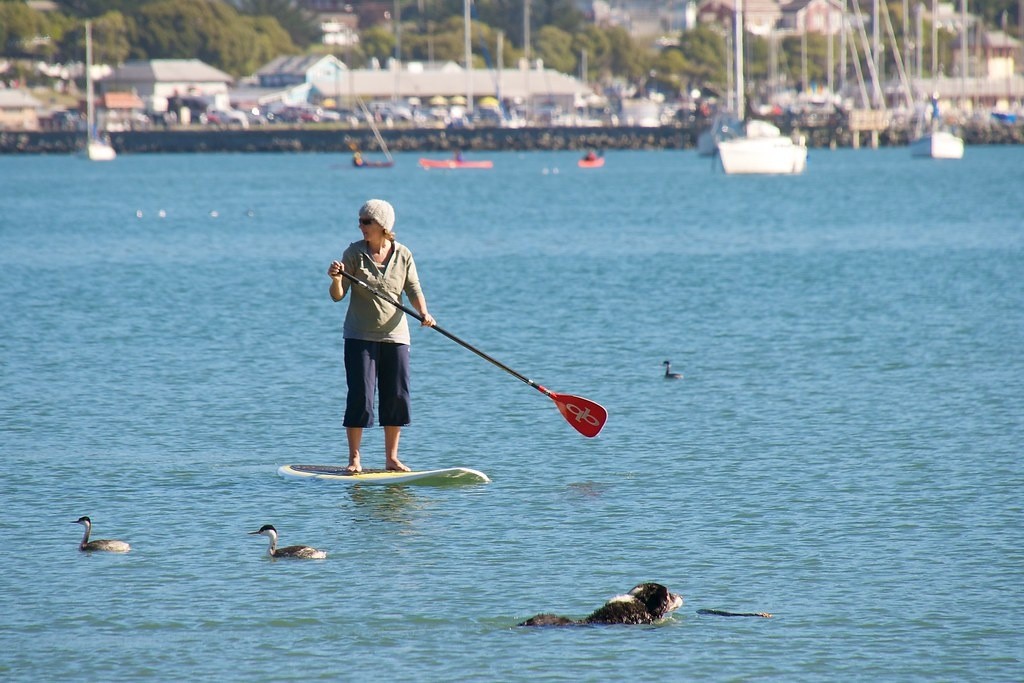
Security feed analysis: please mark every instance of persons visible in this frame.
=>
[328,199,437,473]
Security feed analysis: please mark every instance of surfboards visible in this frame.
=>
[277,464,491,485]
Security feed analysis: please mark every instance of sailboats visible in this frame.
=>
[696,0,1024,178]
[76,16,119,164]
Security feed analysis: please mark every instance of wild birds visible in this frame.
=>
[137,210,220,220]
[69,516,132,552]
[247,525,328,562]
[663,361,684,380]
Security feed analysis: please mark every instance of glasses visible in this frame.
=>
[359,218,375,225]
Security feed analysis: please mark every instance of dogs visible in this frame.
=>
[515,583,683,627]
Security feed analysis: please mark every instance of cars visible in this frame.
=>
[200,91,426,123]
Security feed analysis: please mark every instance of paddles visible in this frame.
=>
[331,266,609,438]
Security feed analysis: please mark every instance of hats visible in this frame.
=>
[359,199,395,231]
